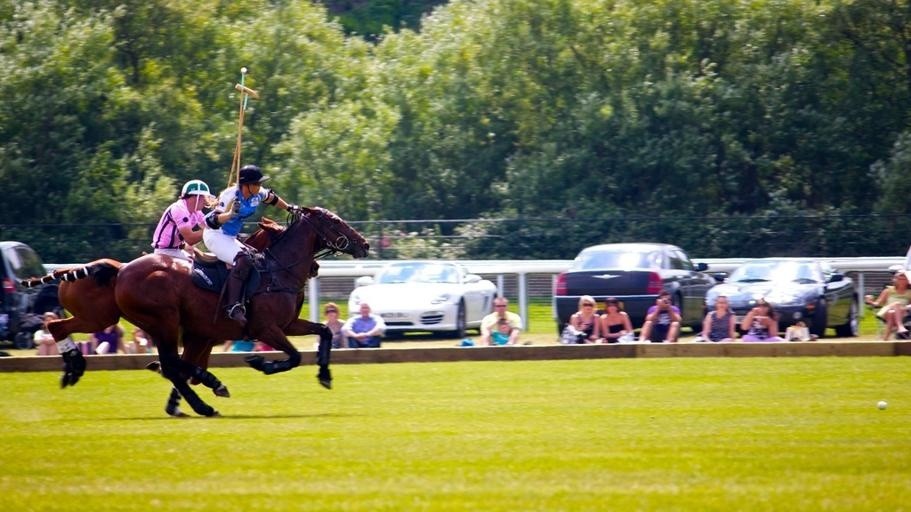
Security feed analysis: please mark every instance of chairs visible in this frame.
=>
[875,281,910,341]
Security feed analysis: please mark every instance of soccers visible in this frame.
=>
[878,401,887,411]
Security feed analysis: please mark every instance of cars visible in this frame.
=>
[0,233,61,353]
[549,240,718,338]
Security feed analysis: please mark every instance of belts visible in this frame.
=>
[172,243,185,250]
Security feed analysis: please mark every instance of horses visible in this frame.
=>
[12,217,321,418]
[60,205,370,418]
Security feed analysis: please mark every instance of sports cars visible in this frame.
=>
[702,258,863,340]
[345,259,500,339]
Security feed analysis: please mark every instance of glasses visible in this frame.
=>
[584,303,592,306]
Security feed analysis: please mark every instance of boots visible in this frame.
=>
[227,269,247,322]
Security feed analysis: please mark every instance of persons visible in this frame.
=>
[34,311,276,357]
[562,290,782,344]
[864,272,911,341]
[488,318,511,347]
[317,301,349,349]
[339,301,387,348]
[151,180,208,259]
[202,165,299,327]
[479,296,522,349]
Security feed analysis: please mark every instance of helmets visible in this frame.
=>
[180,179,211,198]
[238,164,272,185]
